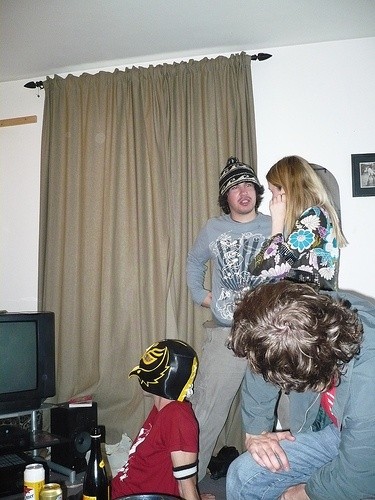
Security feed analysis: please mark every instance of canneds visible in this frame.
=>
[23,463,45,500]
[39,483,63,500]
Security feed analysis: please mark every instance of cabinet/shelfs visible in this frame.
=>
[0,402,75,500]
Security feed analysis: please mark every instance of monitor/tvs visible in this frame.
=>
[0,308,56,415]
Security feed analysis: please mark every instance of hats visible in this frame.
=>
[217,156,263,196]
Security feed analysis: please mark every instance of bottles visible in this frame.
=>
[83,427,110,500]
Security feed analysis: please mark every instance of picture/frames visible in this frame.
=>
[351,153,375,197]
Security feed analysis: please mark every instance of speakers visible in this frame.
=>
[50,399,99,474]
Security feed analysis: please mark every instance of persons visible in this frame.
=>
[245,156,347,291]
[108,338,216,500]
[225,280,375,500]
[184,155,272,486]
